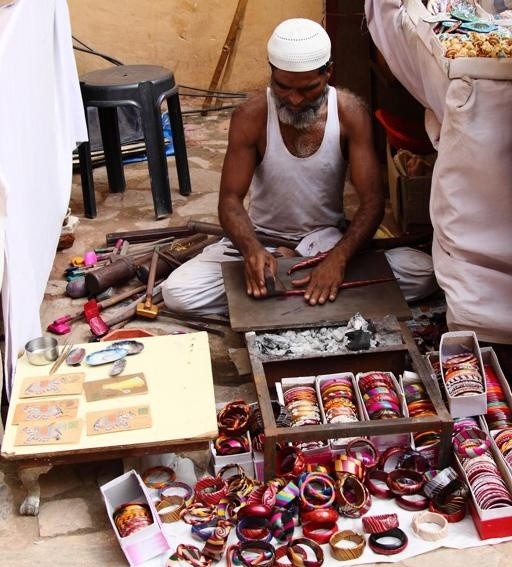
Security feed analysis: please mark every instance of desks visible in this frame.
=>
[15,438,211,517]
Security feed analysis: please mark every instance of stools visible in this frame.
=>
[78,65,192,220]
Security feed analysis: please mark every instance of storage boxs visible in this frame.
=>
[386,139,431,234]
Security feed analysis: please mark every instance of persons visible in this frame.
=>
[161,17,441,316]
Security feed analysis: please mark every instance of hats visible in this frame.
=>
[267,18,332,73]
